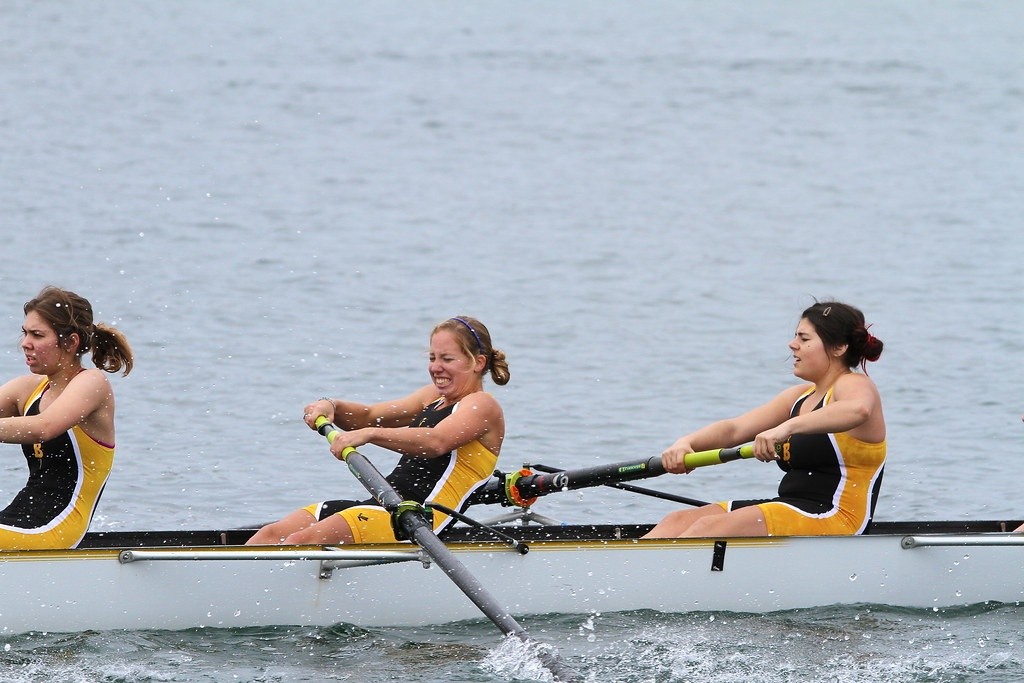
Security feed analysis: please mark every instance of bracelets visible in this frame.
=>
[318,398,337,414]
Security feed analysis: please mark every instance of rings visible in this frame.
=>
[331,450,335,453]
[304,414,308,417]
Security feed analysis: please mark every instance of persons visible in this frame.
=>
[639,303,886,538]
[0,289,133,553]
[244,315,509,545]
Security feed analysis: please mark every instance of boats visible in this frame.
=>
[1,519,1024,632]
